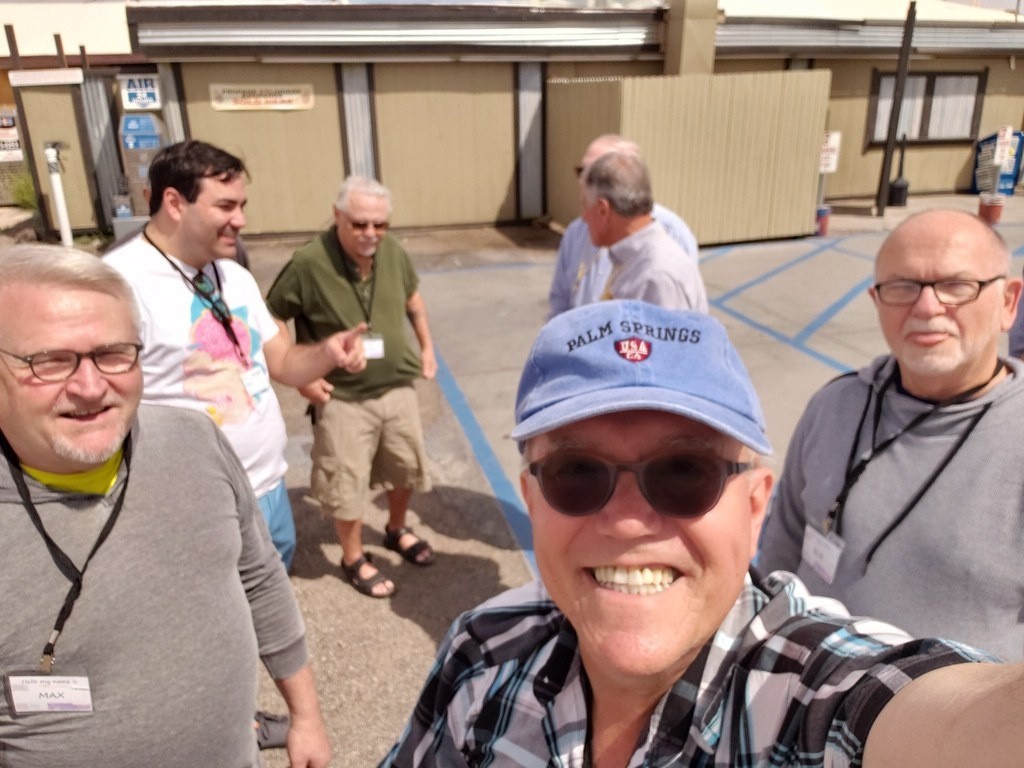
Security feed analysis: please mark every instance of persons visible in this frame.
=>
[549,131,709,313]
[375,301,1024,768]
[99,140,373,578]
[750,207,1024,663]
[0,243,332,768]
[264,175,440,598]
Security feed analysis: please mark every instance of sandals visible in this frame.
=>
[342,554,398,598]
[384,524,437,565]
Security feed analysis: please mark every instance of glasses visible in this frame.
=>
[529,448,754,519]
[875,274,1005,304]
[339,210,388,232]
[0,342,144,382]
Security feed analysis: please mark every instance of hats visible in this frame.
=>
[509,299,777,456]
